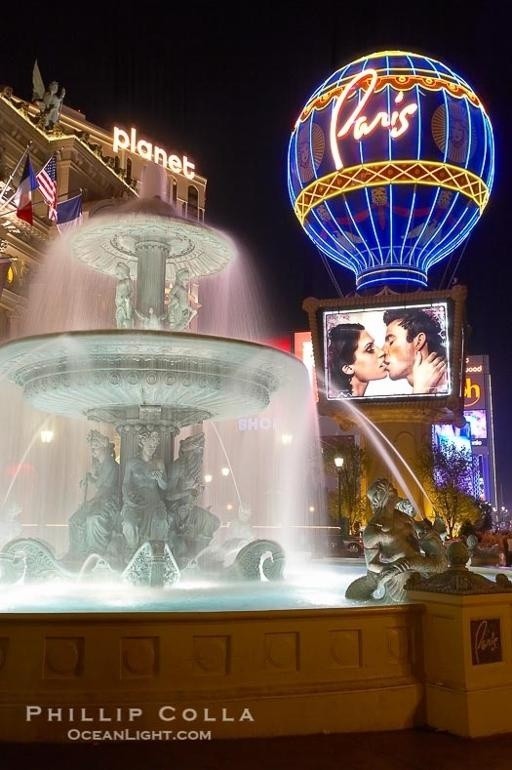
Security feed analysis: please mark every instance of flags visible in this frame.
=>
[15,153,40,228]
[56,194,83,235]
[37,157,60,224]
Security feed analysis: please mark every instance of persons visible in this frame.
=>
[35,81,67,132]
[328,322,445,394]
[381,308,448,396]
[68,430,123,559]
[164,432,222,555]
[502,532,510,567]
[118,431,171,552]
[506,533,511,565]
[363,478,423,605]
